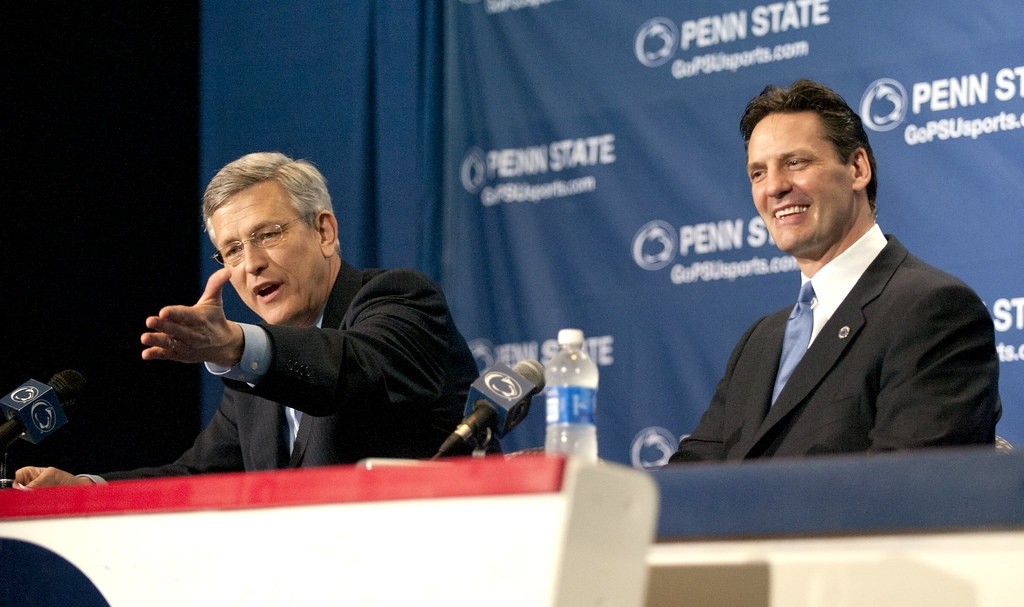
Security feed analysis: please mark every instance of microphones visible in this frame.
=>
[0,369,85,452]
[439,358,547,456]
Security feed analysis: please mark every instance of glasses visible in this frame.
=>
[211,213,308,265]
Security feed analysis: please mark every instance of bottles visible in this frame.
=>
[544,329,600,458]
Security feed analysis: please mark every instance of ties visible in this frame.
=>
[770,279,815,421]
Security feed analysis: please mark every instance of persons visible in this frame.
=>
[12,153,501,489]
[660,80,1003,471]
